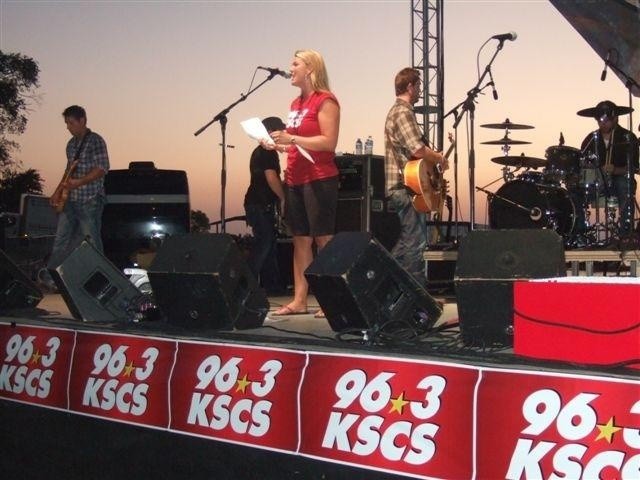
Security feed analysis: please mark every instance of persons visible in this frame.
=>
[260,49,340,318]
[245,117,293,299]
[384,68,449,310]
[43,105,110,281]
[570,100,639,248]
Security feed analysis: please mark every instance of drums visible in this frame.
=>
[488,179,576,244]
[544,144,583,176]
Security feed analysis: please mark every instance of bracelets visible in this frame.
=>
[281,144,286,153]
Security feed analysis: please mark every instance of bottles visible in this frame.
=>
[368,136,373,146]
[356,138,362,155]
[365,139,371,154]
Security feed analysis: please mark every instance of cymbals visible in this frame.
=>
[479,134,533,145]
[489,155,547,168]
[479,118,535,130]
[577,100,634,116]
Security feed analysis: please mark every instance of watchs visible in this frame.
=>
[291,135,295,146]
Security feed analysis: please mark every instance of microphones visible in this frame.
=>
[601,51,611,82]
[259,66,290,78]
[492,31,519,42]
[488,69,498,100]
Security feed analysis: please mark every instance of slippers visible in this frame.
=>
[271,305,310,316]
[314,310,327,319]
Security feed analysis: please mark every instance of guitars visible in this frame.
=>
[403,133,456,214]
[48,159,82,210]
